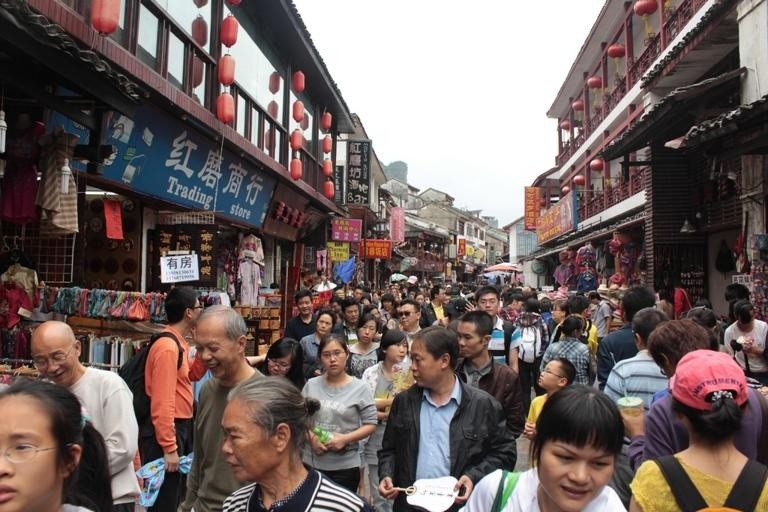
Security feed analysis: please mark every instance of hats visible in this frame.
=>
[670,349,749,414]
[407,275,419,286]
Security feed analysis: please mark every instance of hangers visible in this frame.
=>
[0,357,43,380]
[74,325,150,346]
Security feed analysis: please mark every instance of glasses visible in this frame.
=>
[33,339,78,367]
[399,311,419,317]
[0,442,77,465]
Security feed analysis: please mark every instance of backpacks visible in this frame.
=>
[515,317,543,365]
[119,331,187,425]
[652,452,768,512]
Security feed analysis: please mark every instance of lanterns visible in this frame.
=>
[561,186,569,195]
[572,99,584,121]
[634,1,659,35]
[586,76,602,102]
[573,174,585,186]
[606,43,625,73]
[560,120,570,131]
[589,158,603,171]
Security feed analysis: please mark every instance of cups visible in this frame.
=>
[618,395,644,420]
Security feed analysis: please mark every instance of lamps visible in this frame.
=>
[696,203,712,219]
[60,82,71,195]
[679,206,694,232]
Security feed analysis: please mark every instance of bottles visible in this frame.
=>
[313,424,342,450]
